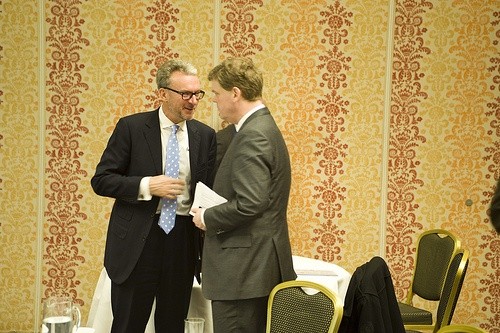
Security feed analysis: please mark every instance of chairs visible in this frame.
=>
[266,229,488,333]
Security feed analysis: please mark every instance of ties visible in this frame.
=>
[157,124,179,234]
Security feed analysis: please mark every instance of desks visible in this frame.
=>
[86,256,352,333]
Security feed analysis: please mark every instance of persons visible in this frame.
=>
[91,59,219,333]
[190,57,298,333]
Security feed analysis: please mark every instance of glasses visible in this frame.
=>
[160,86,205,101]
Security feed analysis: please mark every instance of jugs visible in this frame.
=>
[41,297,80,333]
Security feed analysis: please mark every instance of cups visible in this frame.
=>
[184,318,204,333]
